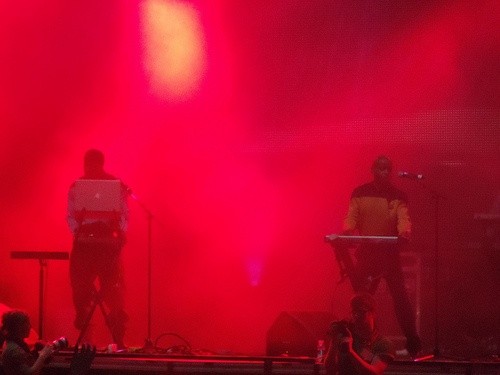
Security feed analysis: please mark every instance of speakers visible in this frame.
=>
[265,309,335,357]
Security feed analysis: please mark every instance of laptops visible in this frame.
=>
[76,179,122,211]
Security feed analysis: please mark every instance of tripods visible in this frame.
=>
[129,196,173,357]
[413,179,467,362]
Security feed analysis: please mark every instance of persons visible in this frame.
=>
[67,149,129,351]
[0,311,58,375]
[344,156,423,356]
[68,344,96,375]
[325,291,395,375]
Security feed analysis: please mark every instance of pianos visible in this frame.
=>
[326,235,399,244]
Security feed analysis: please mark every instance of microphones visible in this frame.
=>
[398,171,424,180]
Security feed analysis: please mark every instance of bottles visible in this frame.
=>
[317,340,326,362]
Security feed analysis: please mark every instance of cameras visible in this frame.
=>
[47,336,68,353]
